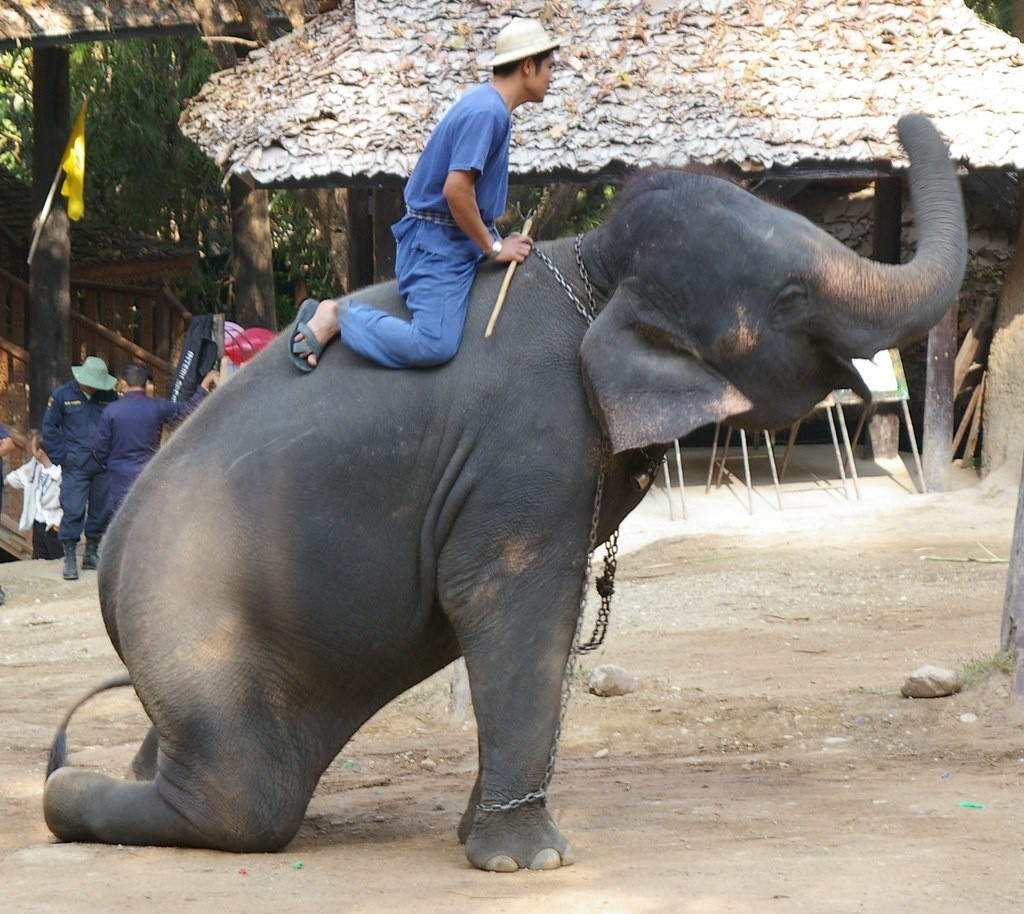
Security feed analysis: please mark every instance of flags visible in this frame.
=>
[60,101,85,222]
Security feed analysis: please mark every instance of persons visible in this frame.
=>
[0,421,16,606]
[42,356,121,580]
[288,18,568,373]
[3,429,65,559]
[95,357,219,516]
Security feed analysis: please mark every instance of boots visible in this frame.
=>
[61,541,78,580]
[81,536,100,569]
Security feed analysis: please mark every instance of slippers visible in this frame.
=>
[287,298,326,372]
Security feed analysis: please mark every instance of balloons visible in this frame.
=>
[224,321,245,364]
[225,328,276,365]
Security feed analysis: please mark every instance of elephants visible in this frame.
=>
[41,113,969,875]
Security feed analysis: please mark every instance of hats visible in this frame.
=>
[71,356,118,391]
[488,17,563,66]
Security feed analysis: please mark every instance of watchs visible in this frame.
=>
[487,239,503,258]
